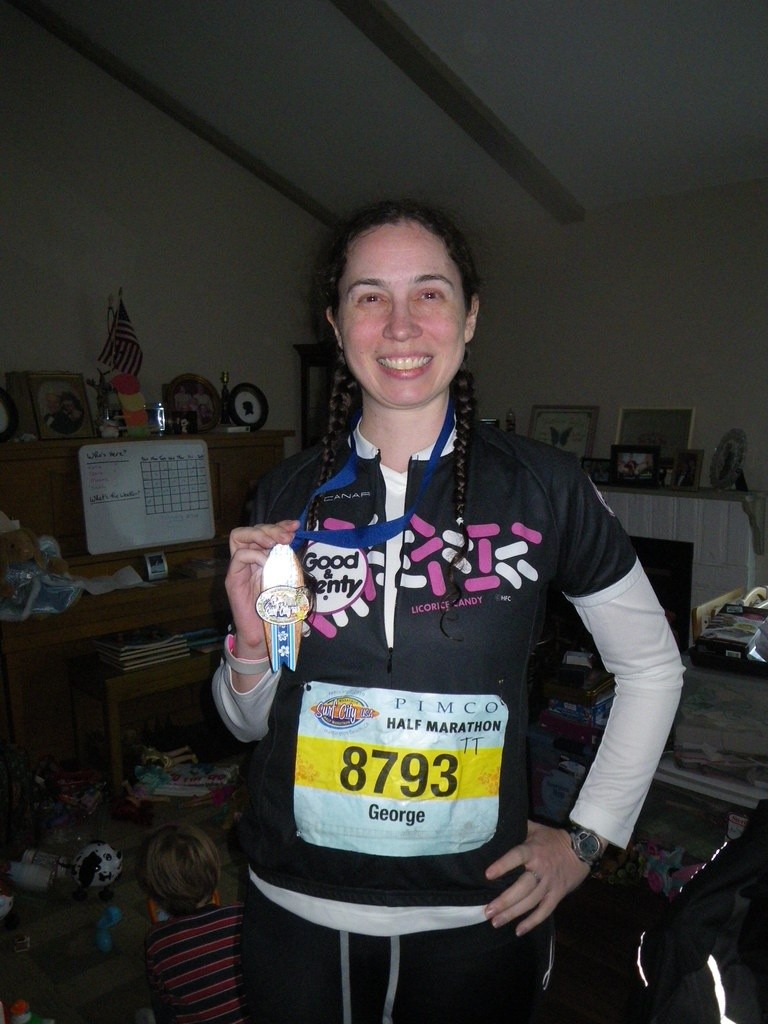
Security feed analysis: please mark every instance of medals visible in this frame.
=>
[255,543,314,673]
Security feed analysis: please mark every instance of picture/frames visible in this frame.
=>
[583,446,662,489]
[166,374,222,434]
[671,449,705,492]
[528,404,601,459]
[230,382,269,432]
[710,429,749,492]
[25,371,97,440]
[616,407,695,472]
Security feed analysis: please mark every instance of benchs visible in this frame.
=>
[62,631,227,800]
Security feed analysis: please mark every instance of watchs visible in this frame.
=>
[556,815,612,874]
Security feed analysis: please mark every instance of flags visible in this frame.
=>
[97,300,143,378]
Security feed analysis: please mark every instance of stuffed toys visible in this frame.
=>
[0,510,87,623]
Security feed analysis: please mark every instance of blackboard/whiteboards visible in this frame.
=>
[78,438,217,556]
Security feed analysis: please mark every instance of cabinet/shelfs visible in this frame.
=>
[0,429,300,770]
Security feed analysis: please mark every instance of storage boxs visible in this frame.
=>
[526,721,599,825]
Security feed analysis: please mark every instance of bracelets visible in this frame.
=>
[223,634,271,675]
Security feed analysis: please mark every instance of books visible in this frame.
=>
[79,626,192,671]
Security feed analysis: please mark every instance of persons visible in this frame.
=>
[42,390,83,433]
[134,823,245,1023]
[210,199,689,1023]
[174,382,215,424]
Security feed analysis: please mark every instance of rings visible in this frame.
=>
[525,869,541,883]
